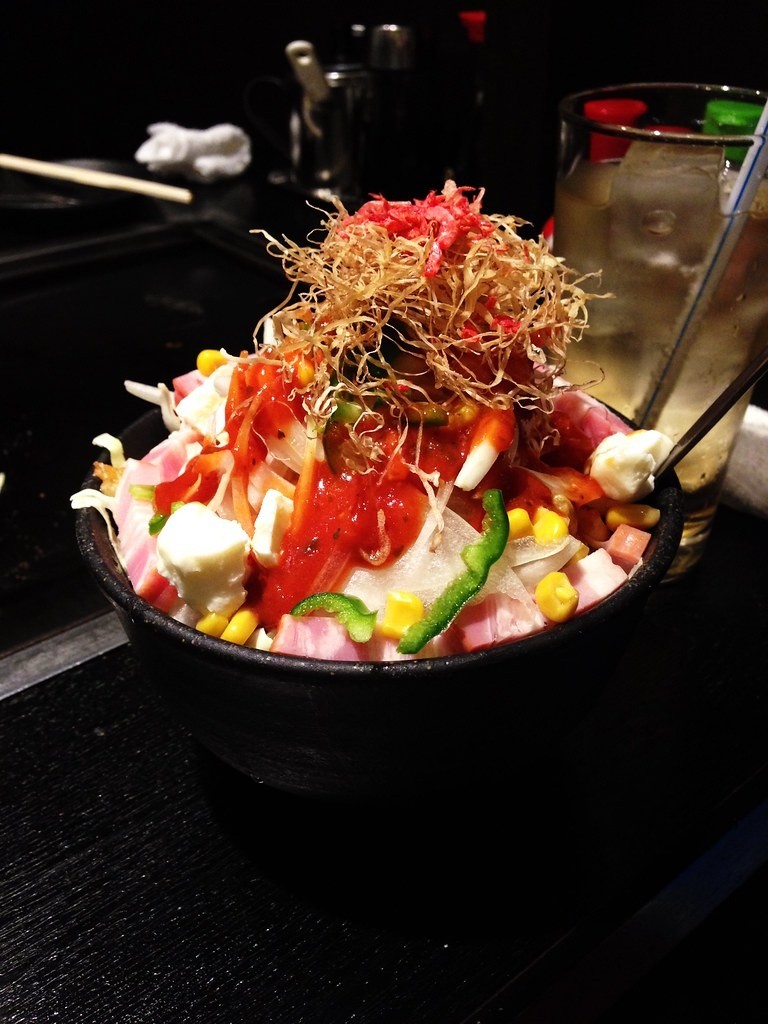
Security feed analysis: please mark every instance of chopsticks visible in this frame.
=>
[0,154,194,205]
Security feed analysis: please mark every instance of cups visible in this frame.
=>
[552,85,767,584]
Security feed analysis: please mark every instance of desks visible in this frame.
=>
[1,375,768,1024]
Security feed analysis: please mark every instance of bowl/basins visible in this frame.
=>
[77,395,684,797]
[0,159,152,223]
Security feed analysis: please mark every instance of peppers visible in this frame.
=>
[130,384,512,652]
